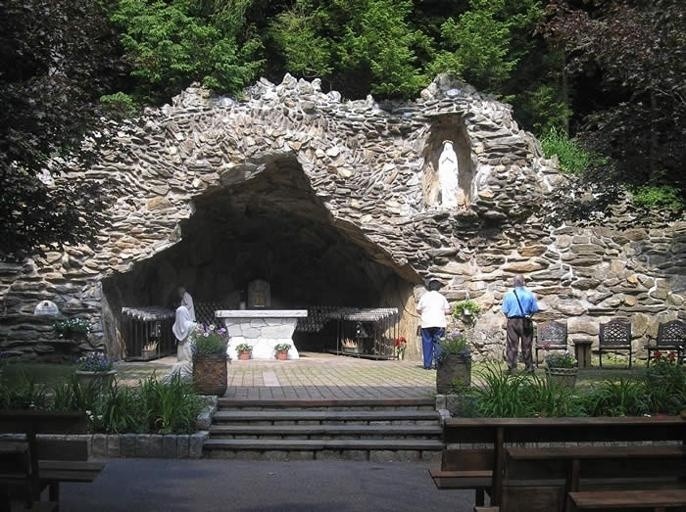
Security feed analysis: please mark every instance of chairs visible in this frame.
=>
[599,319,632,368]
[535,320,568,368]
[644,319,686,368]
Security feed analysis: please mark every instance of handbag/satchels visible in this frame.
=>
[523,316,532,337]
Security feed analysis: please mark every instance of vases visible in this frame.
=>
[192,353,227,397]
[74,369,117,390]
[436,351,472,394]
[64,326,86,340]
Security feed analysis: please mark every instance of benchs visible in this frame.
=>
[428,417,686,512]
[0,410,106,512]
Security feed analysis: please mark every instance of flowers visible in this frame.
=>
[76,352,113,371]
[438,329,468,353]
[191,325,231,354]
[53,316,92,337]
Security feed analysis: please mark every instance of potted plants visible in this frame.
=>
[544,350,579,390]
[235,343,253,360]
[274,343,291,360]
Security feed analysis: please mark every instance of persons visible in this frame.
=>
[416,281,450,370]
[503,275,538,374]
[176,286,196,322]
[439,139,458,208]
